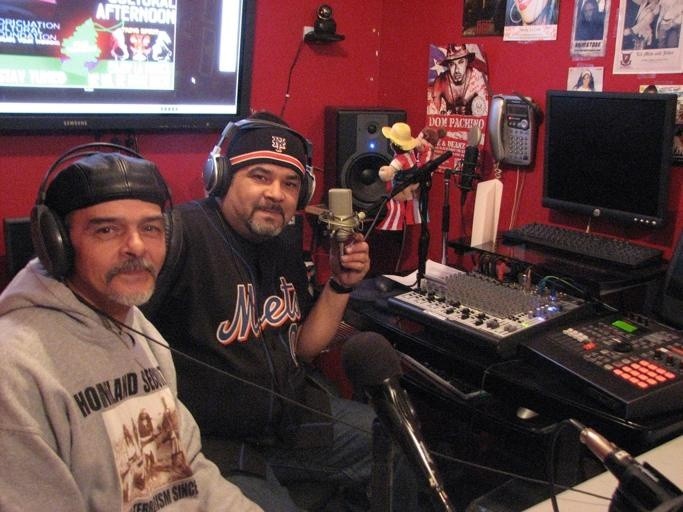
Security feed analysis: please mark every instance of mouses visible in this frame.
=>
[515,405,540,420]
[372,275,394,292]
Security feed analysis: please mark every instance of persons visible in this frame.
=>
[0,150,266,512]
[433,43,489,121]
[507,1,557,26]
[137,108,427,512]
[576,0,605,42]
[621,0,655,47]
[642,85,657,94]
[637,0,683,50]
[564,68,602,93]
[672,125,683,156]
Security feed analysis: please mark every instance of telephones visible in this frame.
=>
[488,94,539,167]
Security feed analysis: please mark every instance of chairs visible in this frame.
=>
[0,216,31,289]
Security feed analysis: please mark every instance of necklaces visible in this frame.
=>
[445,73,466,115]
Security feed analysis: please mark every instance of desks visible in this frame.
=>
[522,434,682,510]
[342,261,681,451]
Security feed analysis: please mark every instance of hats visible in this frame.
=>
[228,112,307,181]
[45,153,169,216]
[439,44,474,66]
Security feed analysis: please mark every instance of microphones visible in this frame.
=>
[568,422,683,512]
[341,331,454,512]
[317,188,365,241]
[461,126,482,191]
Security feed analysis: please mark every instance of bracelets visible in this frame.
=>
[326,276,355,296]
[629,28,634,36]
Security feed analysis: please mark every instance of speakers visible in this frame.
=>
[336,109,407,217]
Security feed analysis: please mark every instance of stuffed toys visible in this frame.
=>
[411,125,446,225]
[374,121,419,232]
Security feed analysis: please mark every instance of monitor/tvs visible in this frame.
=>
[0,0,257,131]
[541,90,678,228]
[653,225,683,325]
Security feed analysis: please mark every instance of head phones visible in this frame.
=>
[204,119,316,210]
[29,141,184,282]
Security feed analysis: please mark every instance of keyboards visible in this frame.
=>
[502,222,663,270]
[385,342,489,399]
